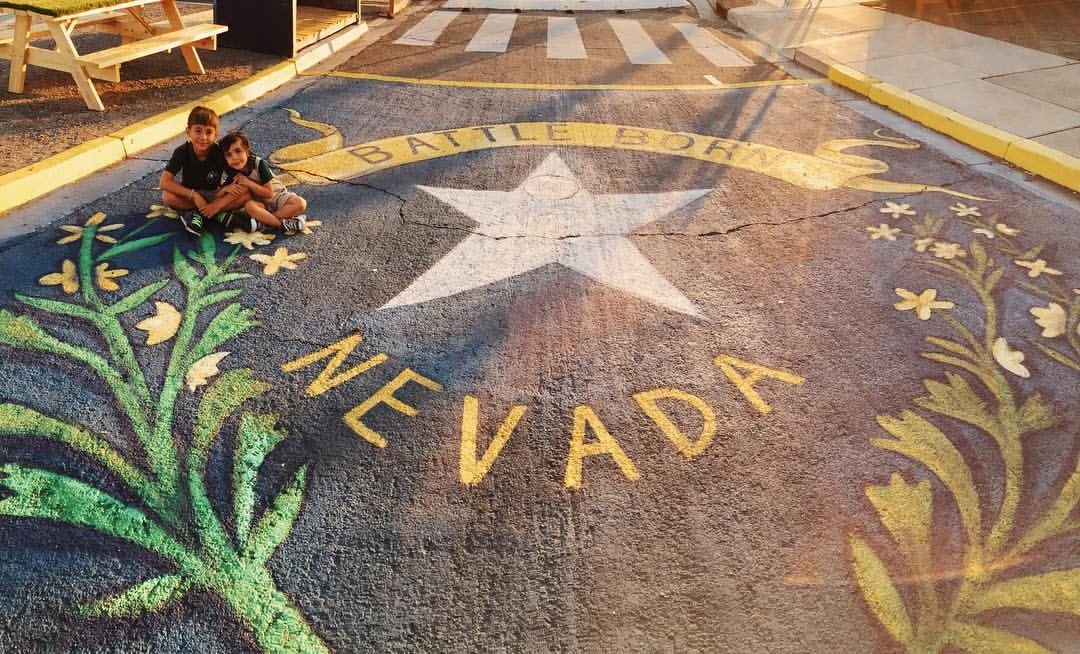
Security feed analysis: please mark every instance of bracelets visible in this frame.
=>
[189,189,194,200]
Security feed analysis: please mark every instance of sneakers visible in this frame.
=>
[180,210,202,236]
[215,211,231,228]
[250,217,263,234]
[282,217,305,232]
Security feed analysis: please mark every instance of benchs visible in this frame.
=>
[0,12,228,82]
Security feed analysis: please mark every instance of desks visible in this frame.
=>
[0,0,206,111]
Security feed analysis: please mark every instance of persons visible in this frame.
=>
[216,132,307,232]
[159,106,251,237]
